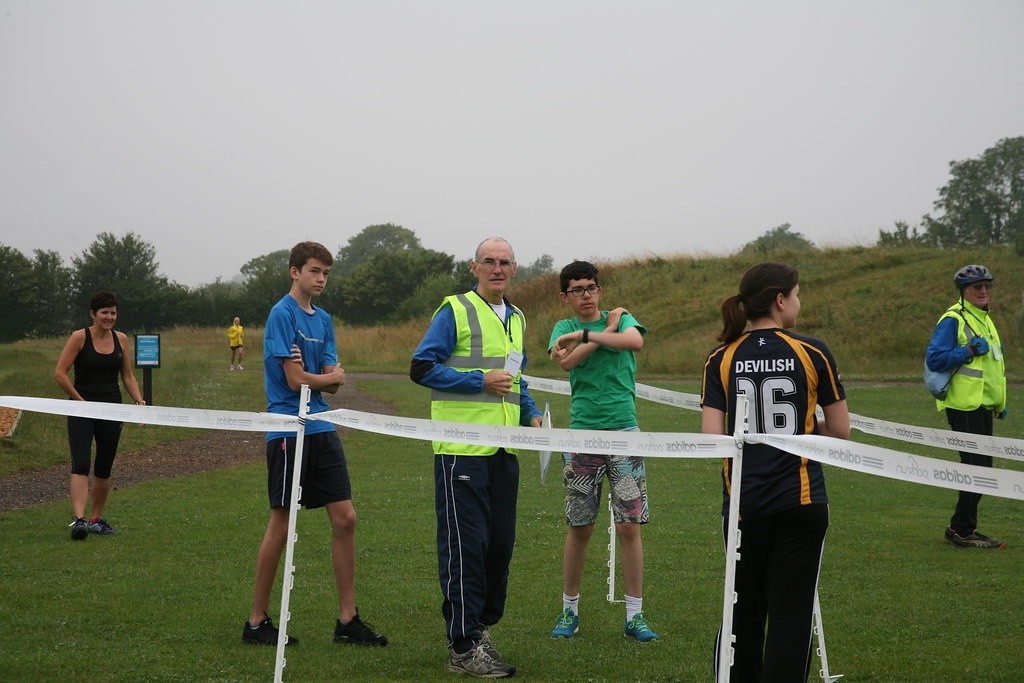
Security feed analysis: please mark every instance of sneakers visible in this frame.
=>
[87,518,114,535]
[332,607,389,647]
[943,526,957,544]
[478,628,506,663]
[70,515,89,540]
[241,611,300,648]
[549,606,580,640]
[951,529,1006,550]
[448,636,517,679]
[624,611,661,642]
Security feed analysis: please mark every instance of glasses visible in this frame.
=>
[969,281,992,289]
[563,284,599,297]
[476,259,515,268]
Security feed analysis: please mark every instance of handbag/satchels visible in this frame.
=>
[923,309,975,401]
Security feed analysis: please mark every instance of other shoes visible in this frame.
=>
[229,365,235,372]
[237,365,244,370]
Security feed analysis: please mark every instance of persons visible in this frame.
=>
[51,292,145,540]
[547,260,659,642]
[926,265,1007,547]
[241,241,387,649]
[701,263,850,682]
[410,237,553,679]
[228,317,244,371]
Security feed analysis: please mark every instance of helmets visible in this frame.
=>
[953,264,993,289]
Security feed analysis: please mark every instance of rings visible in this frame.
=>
[583,329,588,343]
